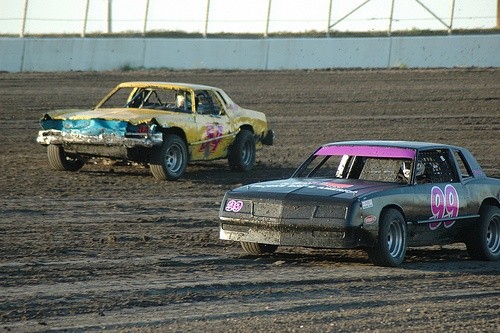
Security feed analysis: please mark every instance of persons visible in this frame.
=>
[402,158,426,184]
[175,94,187,108]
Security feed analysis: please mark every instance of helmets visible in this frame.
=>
[403,160,425,178]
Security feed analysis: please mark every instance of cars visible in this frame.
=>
[36,81,274,182]
[217,140,500,266]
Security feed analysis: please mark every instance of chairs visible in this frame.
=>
[196,96,215,115]
[394,162,426,186]
